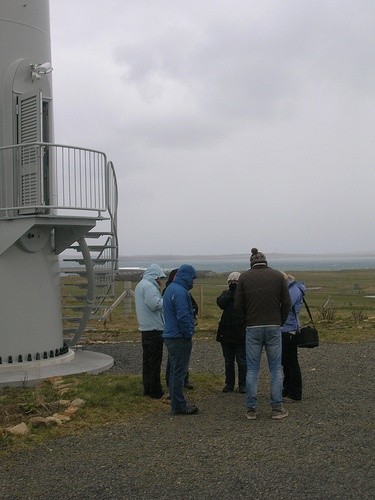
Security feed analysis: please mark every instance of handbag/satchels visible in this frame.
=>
[295,326,319,349]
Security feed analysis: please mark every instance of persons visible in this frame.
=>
[280,272,306,402]
[135,264,198,415]
[234,248,289,419]
[216,272,247,392]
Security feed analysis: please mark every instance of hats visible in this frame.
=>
[227,272,241,286]
[250,248,268,268]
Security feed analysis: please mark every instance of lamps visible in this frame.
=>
[32,61,53,74]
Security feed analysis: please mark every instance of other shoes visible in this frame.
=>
[238,385,247,393]
[161,394,170,399]
[182,406,198,415]
[283,397,295,403]
[223,385,234,393]
[272,408,288,419]
[245,411,256,419]
[185,384,193,389]
